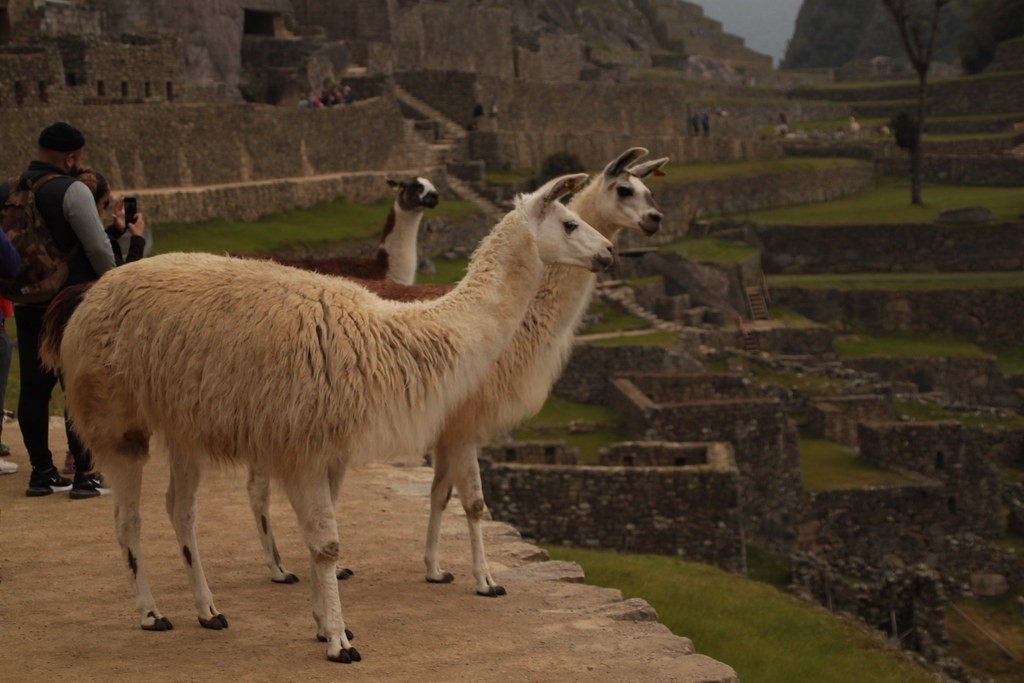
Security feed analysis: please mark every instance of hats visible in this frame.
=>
[38,122,85,151]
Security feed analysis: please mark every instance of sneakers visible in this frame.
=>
[69,471,113,498]
[26,466,73,496]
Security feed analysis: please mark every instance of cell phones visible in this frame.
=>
[124,197,137,229]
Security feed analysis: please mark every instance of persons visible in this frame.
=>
[0,120,146,500]
[472,99,501,132]
[296,77,353,111]
[688,110,712,137]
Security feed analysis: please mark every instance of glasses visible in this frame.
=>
[97,199,110,210]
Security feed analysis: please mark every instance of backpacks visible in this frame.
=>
[0,174,80,304]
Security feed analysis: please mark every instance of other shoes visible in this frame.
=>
[63,450,76,473]
[0,457,18,475]
[0,444,10,456]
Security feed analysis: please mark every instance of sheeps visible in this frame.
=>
[243,146,669,598]
[239,176,440,288]
[42,172,615,663]
